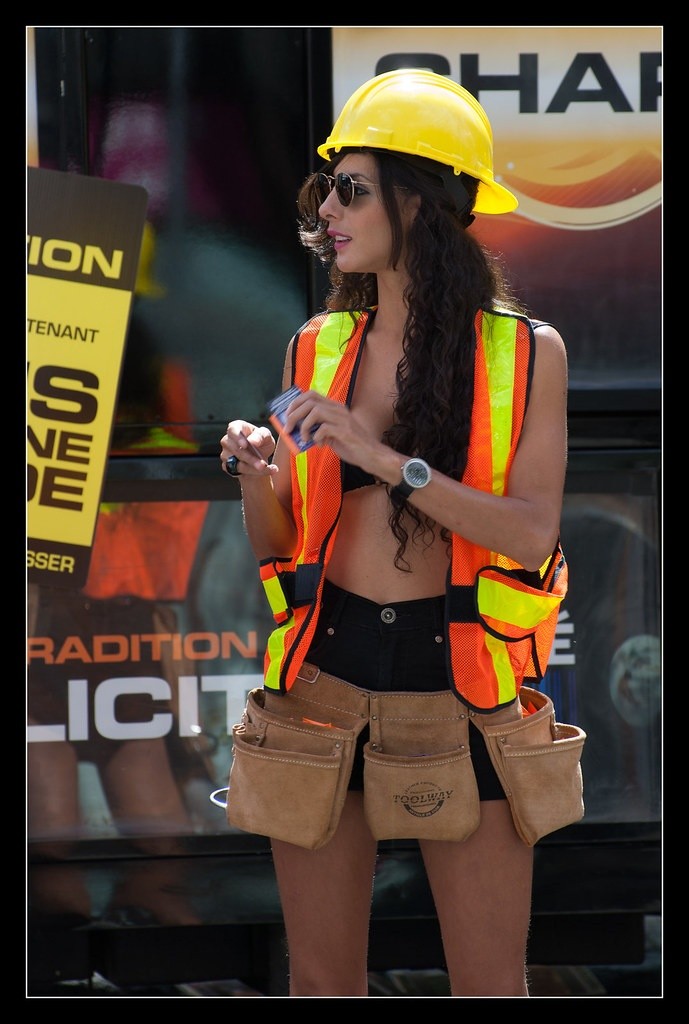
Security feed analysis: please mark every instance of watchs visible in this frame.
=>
[393,457,432,500]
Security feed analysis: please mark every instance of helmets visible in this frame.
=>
[317,69,519,214]
[134,220,169,299]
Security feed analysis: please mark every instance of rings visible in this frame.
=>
[224,454,241,476]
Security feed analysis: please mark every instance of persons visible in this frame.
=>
[28,214,216,929]
[226,65,587,999]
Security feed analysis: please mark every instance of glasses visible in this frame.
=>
[314,173,411,208]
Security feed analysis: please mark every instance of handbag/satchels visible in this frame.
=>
[210,663,587,847]
